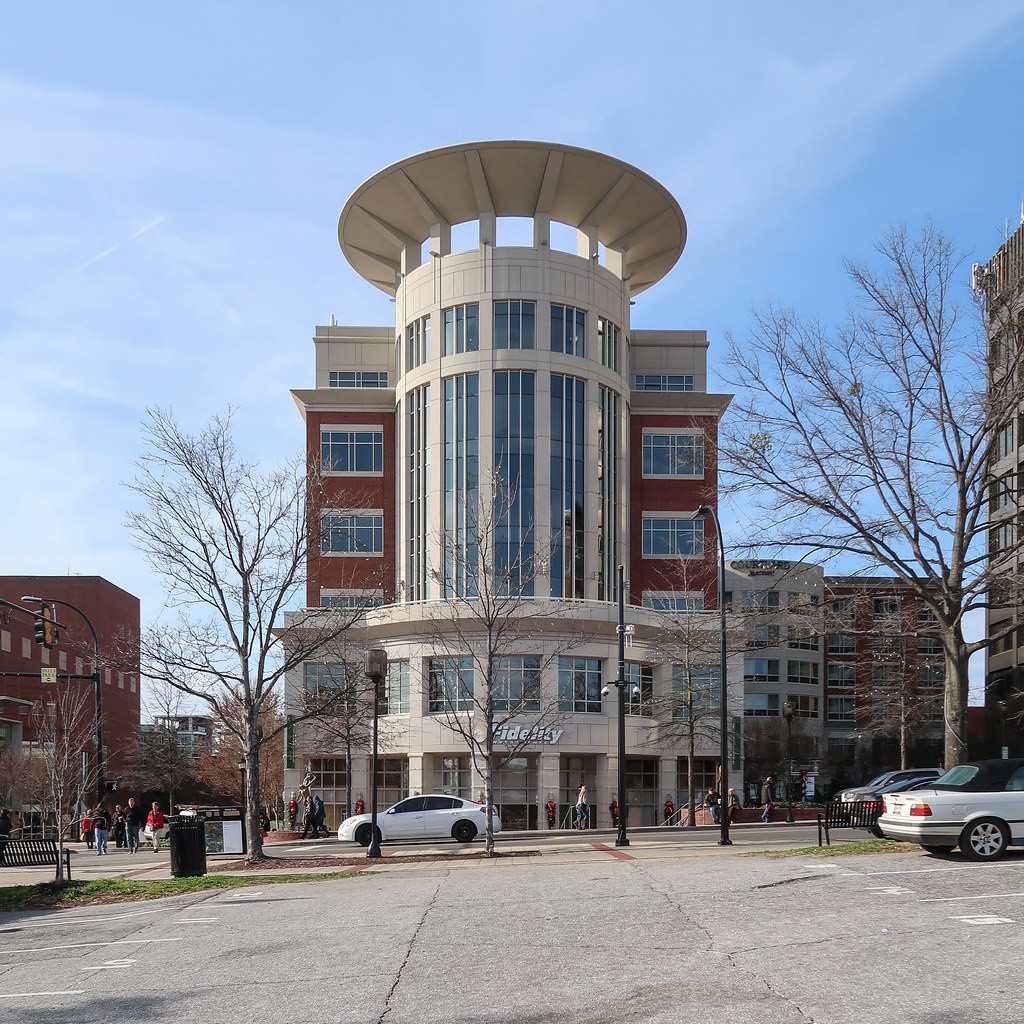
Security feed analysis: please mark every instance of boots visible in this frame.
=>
[573,819,579,828]
[577,820,585,830]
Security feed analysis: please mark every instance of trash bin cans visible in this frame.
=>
[164,815,208,878]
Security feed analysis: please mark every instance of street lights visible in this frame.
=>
[601,680,641,847]
[782,701,796,823]
[688,504,734,847]
[21,596,104,806]
[364,648,388,858]
[237,757,247,854]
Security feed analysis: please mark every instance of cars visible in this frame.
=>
[144,815,180,840]
[337,794,502,848]
[832,773,887,807]
[862,776,940,839]
[876,757,1024,862]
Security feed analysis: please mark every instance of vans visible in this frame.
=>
[179,806,246,823]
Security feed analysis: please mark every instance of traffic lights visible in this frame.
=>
[105,781,118,793]
[34,603,46,644]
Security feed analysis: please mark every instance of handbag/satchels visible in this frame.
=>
[80,832,86,841]
[150,826,155,832]
[577,802,587,811]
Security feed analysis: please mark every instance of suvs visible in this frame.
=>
[841,767,946,822]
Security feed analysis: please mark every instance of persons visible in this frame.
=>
[148,802,164,853]
[90,804,112,856]
[573,782,590,830]
[704,788,721,824]
[728,788,741,823]
[122,798,146,855]
[0,809,13,852]
[259,808,271,832]
[762,777,775,823]
[298,795,331,840]
[111,805,127,849]
[82,808,95,849]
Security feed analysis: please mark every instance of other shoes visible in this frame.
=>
[102,848,106,854]
[129,850,133,854]
[97,852,100,855]
[300,832,330,840]
[761,816,769,823]
[134,847,137,853]
[154,849,158,853]
